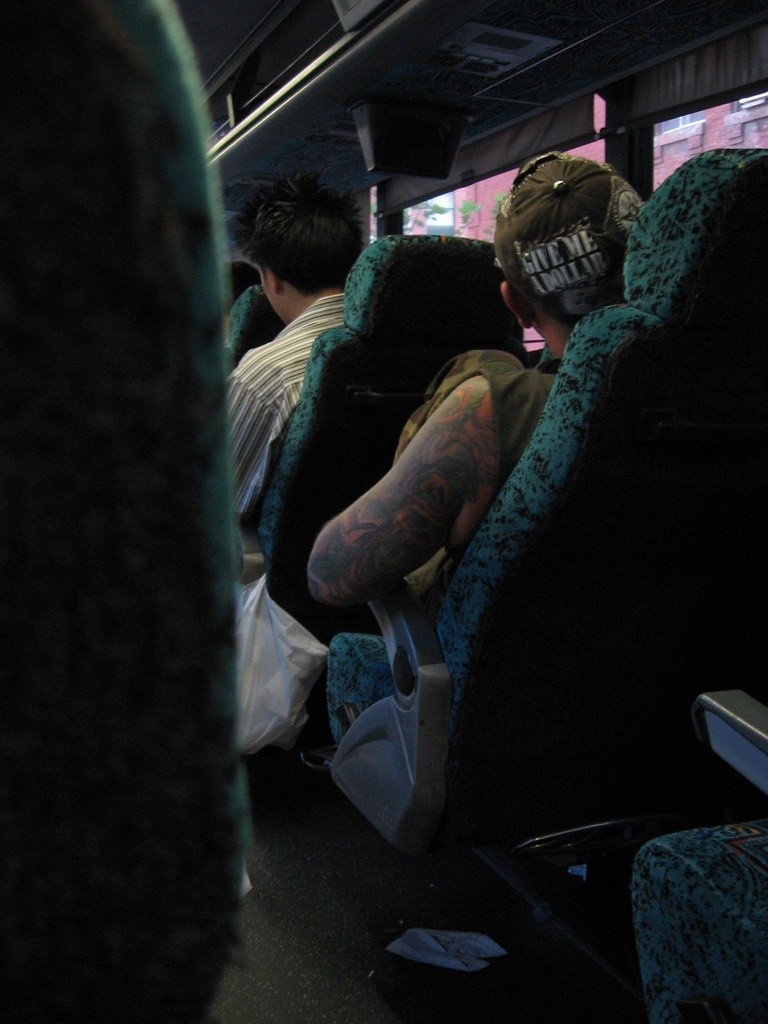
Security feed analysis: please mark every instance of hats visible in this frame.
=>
[494,154,646,326]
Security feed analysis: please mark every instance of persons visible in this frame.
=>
[225,172,365,530]
[221,260,263,345]
[285,158,645,769]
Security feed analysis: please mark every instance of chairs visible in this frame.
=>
[225,144,768,1024]
